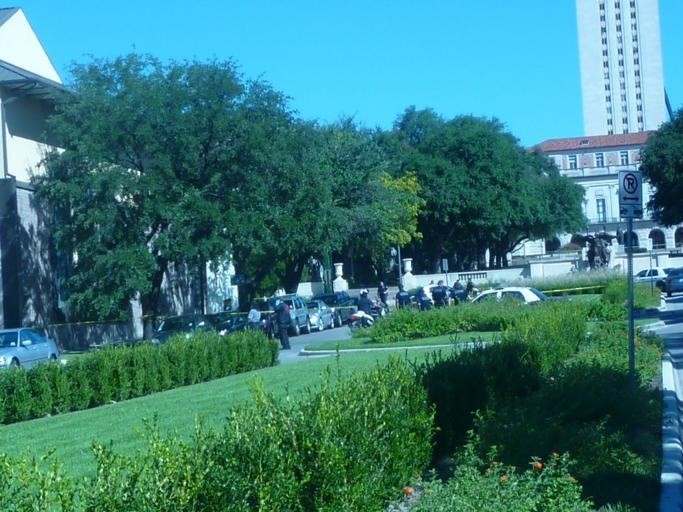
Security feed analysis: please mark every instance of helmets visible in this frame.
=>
[360,289,369,298]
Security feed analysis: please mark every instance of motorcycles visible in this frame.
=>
[348,295,389,333]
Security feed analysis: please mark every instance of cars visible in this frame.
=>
[468,286,572,308]
[632,265,683,297]
[0,327,61,371]
[150,289,358,347]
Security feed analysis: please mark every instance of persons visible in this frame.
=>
[588,244,595,271]
[357,277,474,322]
[274,297,291,349]
[602,243,611,267]
[247,300,261,331]
